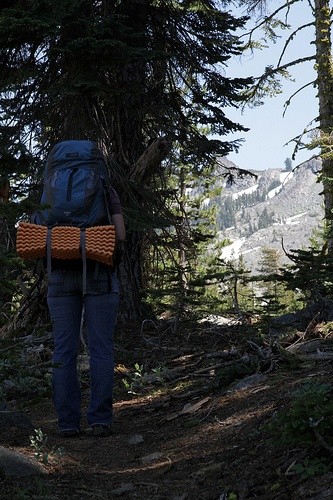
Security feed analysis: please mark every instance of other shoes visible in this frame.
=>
[58,424,112,439]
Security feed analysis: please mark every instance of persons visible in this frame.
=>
[30,131,124,438]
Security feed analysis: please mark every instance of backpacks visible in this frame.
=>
[39,140,108,227]
